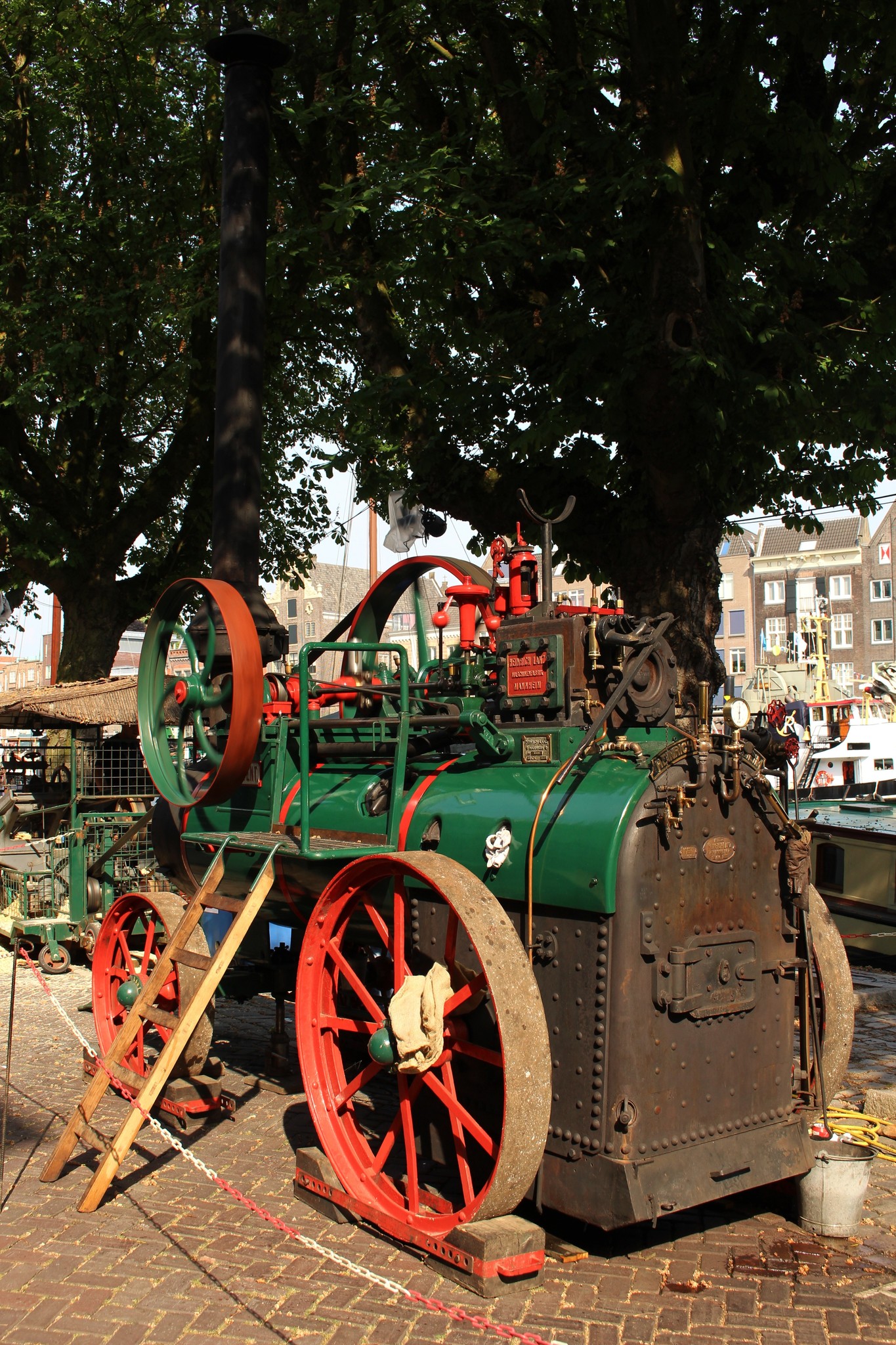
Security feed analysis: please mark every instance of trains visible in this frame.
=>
[0,486,859,1277]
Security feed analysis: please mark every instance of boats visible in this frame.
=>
[755,603,896,804]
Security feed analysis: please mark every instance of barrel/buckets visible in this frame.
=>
[796,1140,878,1238]
